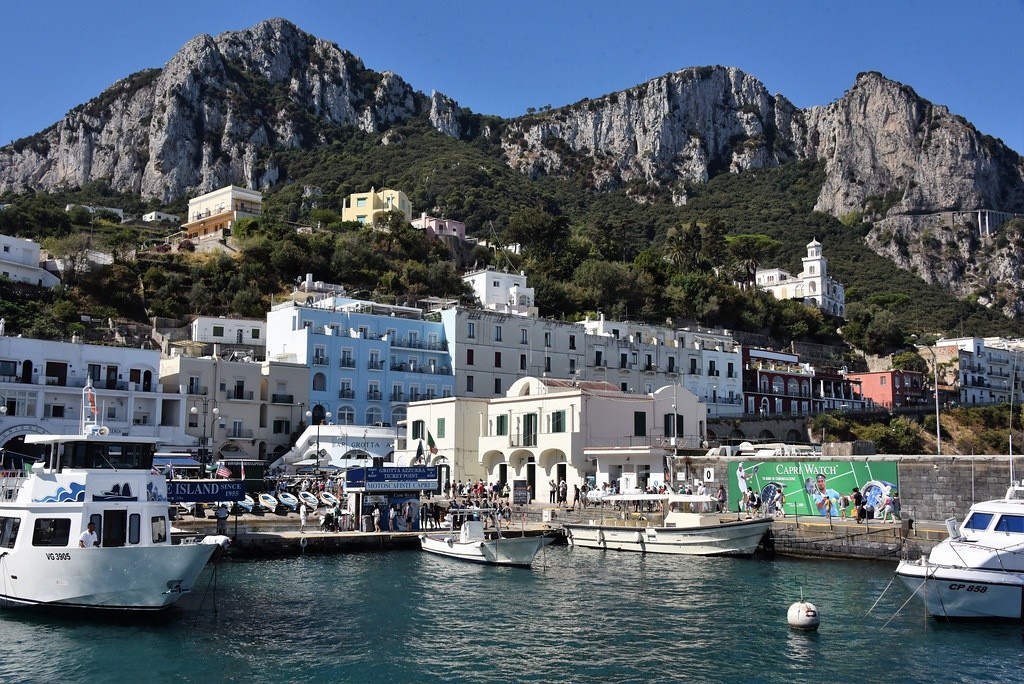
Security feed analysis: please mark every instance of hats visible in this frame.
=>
[748,487,751,491]
[826,496,829,498]
[738,463,742,468]
[816,474,826,479]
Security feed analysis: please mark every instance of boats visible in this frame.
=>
[320,491,340,507]
[179,501,196,514]
[561,481,775,558]
[257,493,278,512]
[237,492,254,512]
[214,501,234,514]
[894,349,1024,624]
[277,491,299,510]
[0,370,231,629]
[299,491,320,511]
[419,484,555,568]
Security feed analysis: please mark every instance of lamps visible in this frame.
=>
[626,457,630,461]
[561,455,564,459]
[585,455,588,462]
[519,457,524,464]
[500,452,503,455]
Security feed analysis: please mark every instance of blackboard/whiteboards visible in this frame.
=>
[514,480,527,504]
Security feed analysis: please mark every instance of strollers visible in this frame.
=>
[320,513,334,531]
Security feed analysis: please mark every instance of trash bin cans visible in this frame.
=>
[362,515,374,532]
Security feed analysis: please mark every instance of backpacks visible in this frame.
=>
[756,498,761,507]
[749,491,755,502]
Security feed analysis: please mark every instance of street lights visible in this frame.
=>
[190,394,219,479]
[911,334,941,454]
[651,363,677,456]
[306,401,332,486]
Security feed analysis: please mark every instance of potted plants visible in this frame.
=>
[442,339,450,351]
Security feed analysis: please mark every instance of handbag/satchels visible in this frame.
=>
[860,496,867,505]
[782,494,786,503]
[845,499,849,506]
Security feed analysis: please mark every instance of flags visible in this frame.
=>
[216,463,233,477]
[242,459,246,481]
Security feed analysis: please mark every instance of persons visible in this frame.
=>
[813,474,851,517]
[405,501,414,531]
[215,504,230,534]
[421,478,532,531]
[736,463,754,511]
[548,476,728,513]
[742,486,787,518]
[371,502,382,532]
[823,488,903,525]
[264,471,346,534]
[388,503,395,533]
[79,522,100,548]
